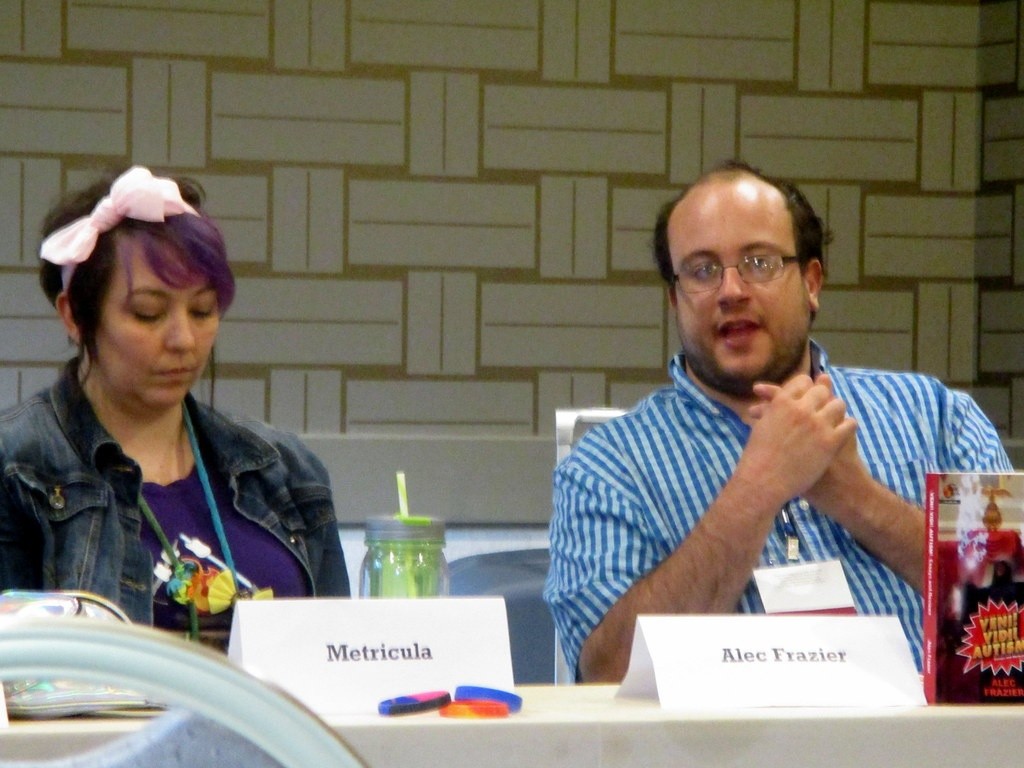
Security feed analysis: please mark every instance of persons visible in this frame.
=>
[0,166,351,660]
[541,159,1015,686]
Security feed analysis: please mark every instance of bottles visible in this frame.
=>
[360,517,449,597]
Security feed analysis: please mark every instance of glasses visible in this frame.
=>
[671,254,809,293]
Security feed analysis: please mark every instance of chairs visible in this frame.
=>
[553,407,625,685]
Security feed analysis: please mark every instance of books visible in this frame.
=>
[924,472,1024,705]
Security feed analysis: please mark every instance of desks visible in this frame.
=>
[0,673,1024,768]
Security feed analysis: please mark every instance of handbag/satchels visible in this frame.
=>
[0,590,171,717]
